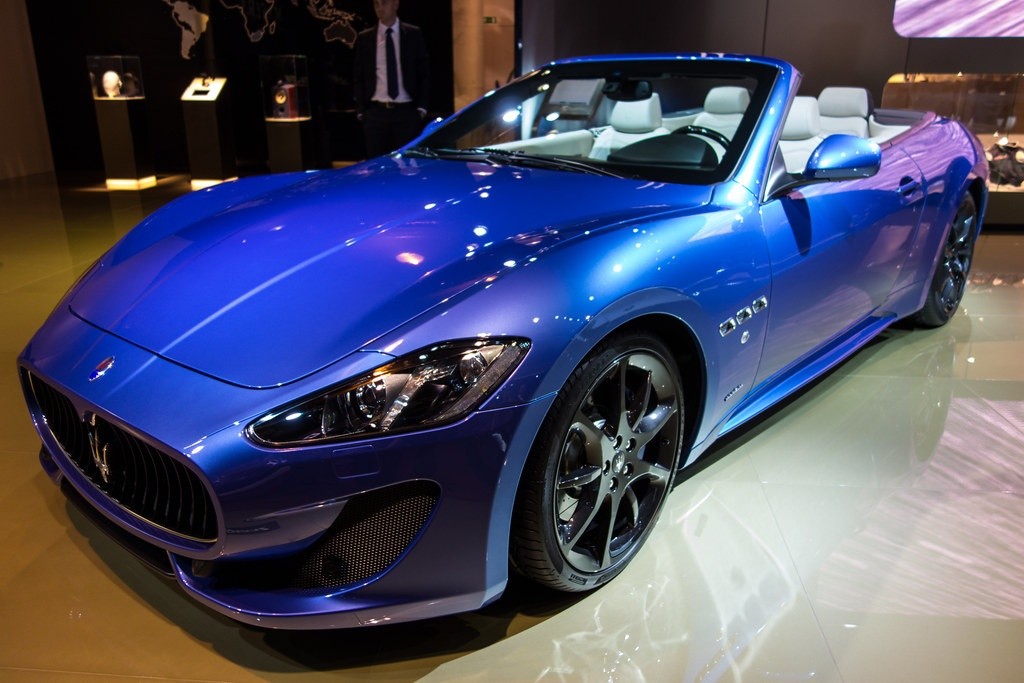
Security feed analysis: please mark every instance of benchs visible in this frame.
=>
[688,87,873,141]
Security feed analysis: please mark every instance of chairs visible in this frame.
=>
[587,93,670,164]
[779,97,824,173]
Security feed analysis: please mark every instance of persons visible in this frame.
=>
[351,0,428,153]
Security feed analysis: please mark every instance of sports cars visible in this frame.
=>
[17,52,989,628]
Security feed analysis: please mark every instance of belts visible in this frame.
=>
[367,102,417,110]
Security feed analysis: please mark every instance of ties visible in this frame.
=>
[385,27,399,100]
